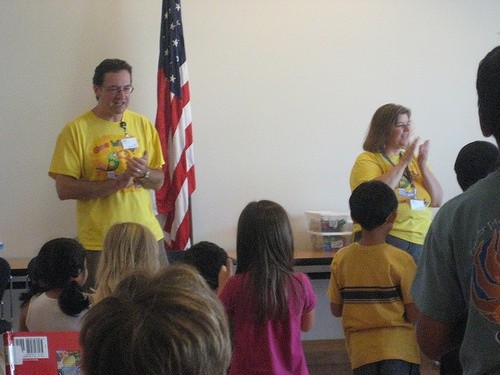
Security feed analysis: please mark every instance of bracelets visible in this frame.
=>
[139,171,150,181]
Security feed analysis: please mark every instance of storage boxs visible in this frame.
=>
[309,232,353,252]
[304,210,349,231]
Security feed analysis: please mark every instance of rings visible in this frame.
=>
[137,170,141,172]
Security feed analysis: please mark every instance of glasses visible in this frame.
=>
[100,84,134,95]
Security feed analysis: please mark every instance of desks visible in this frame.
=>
[4,252,336,290]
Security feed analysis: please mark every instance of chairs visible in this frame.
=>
[0,258,13,336]
[3,331,85,375]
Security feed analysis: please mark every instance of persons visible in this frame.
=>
[48,59,170,294]
[176,200,315,375]
[455,141,499,192]
[410,45,500,375]
[19,238,94,333]
[79,264,231,375]
[76,222,160,331]
[349,103,444,265]
[326,181,423,375]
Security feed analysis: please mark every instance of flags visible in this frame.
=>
[155,0,196,252]
